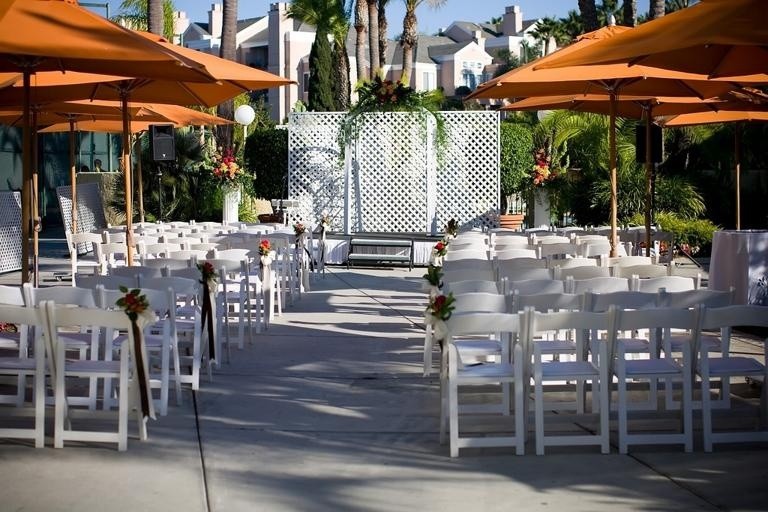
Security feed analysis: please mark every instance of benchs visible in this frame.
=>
[347,237,414,272]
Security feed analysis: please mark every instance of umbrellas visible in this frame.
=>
[654,110,768,234]
[464,14,767,267]
[534,0,767,78]
[0,0,222,290]
[0,30,298,267]
[37,120,160,222]
[11,102,238,266]
[571,97,728,253]
[501,95,727,257]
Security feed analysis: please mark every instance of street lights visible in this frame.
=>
[235,105,256,141]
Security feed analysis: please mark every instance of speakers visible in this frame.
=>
[635,121,662,163]
[148,125,174,161]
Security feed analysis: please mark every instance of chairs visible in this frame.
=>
[439,226,768,457]
[1,219,312,452]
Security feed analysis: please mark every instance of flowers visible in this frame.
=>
[256,239,273,256]
[321,215,331,225]
[636,241,677,258]
[530,147,562,186]
[434,239,450,257]
[115,285,150,315]
[680,242,701,256]
[372,79,407,108]
[212,149,257,198]
[445,219,459,237]
[422,263,445,290]
[425,291,456,321]
[196,262,218,282]
[292,223,307,235]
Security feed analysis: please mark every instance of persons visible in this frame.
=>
[94,159,105,173]
